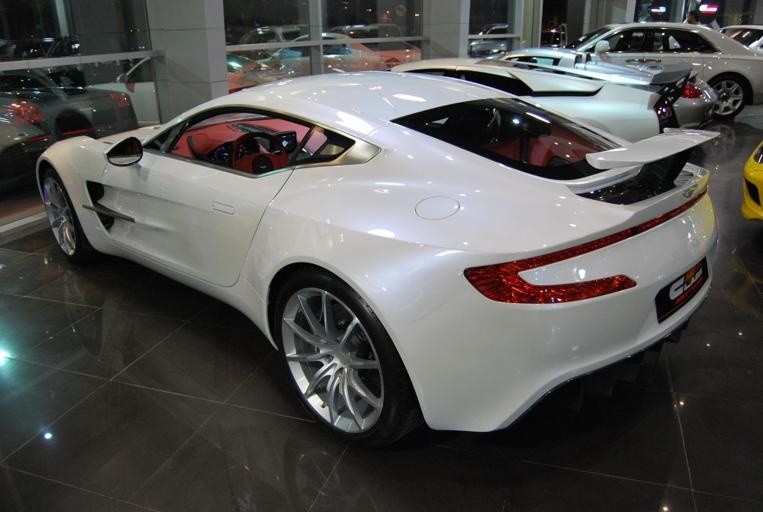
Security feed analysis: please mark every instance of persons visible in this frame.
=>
[683,11,701,25]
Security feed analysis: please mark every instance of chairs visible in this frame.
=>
[615,34,642,51]
